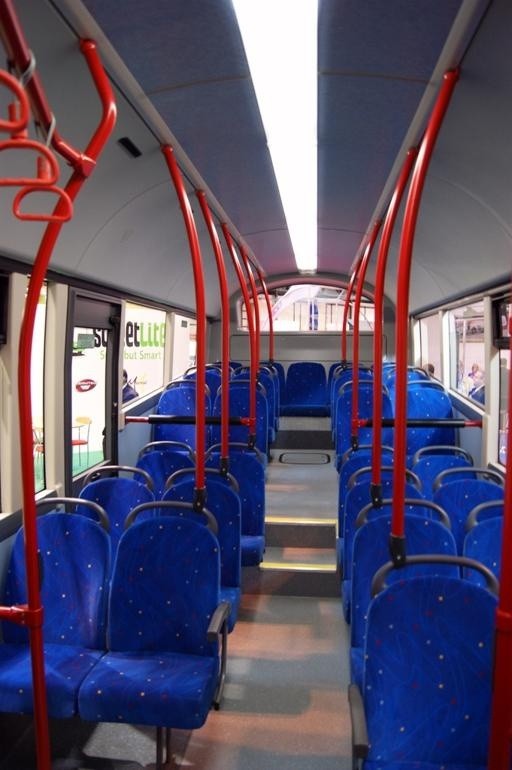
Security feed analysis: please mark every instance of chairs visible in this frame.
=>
[336,445,506,768]
[329,363,458,443]
[1,445,265,767]
[144,367,283,446]
[285,362,330,417]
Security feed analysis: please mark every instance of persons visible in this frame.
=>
[457,359,485,405]
[122,368,139,403]
[423,364,434,377]
[499,357,508,430]
